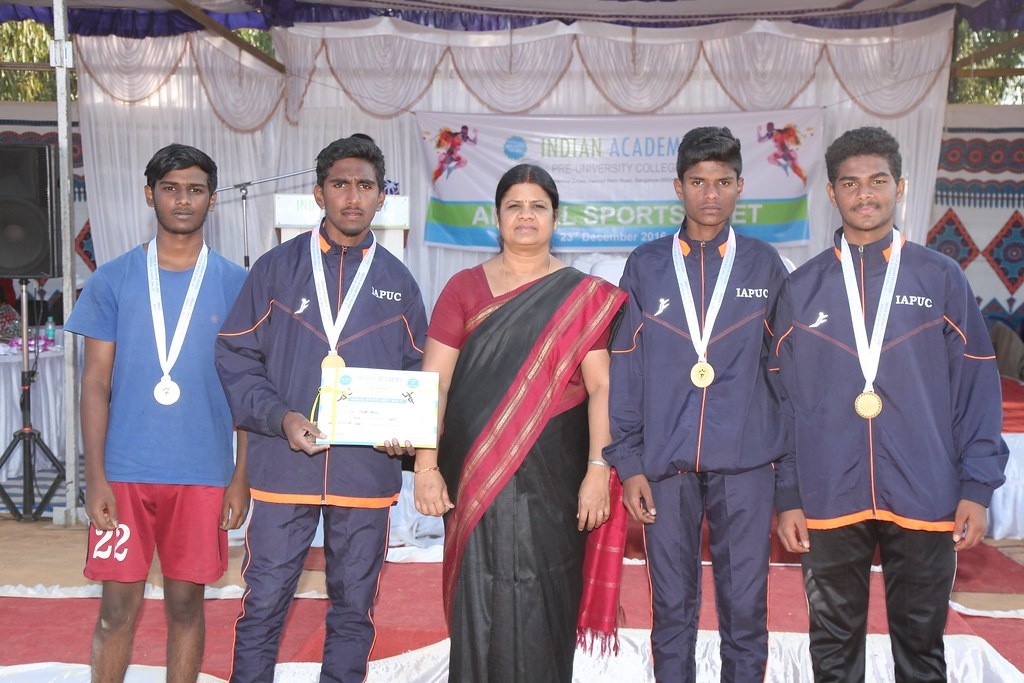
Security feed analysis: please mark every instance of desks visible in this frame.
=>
[0,324,84,483]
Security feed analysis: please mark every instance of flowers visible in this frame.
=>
[0,303,56,356]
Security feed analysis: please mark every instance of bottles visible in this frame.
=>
[44,316,56,347]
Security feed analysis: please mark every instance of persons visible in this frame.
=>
[62,143,251,683]
[411,164,630,683]
[601,127,789,683]
[214,133,429,683]
[768,127,1011,683]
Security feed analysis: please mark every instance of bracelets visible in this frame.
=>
[588,459,611,468]
[415,467,440,475]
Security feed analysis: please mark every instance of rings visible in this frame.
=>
[597,511,603,514]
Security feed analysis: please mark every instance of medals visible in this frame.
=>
[690,362,715,388]
[153,381,180,405]
[855,392,882,419]
[321,354,346,368]
